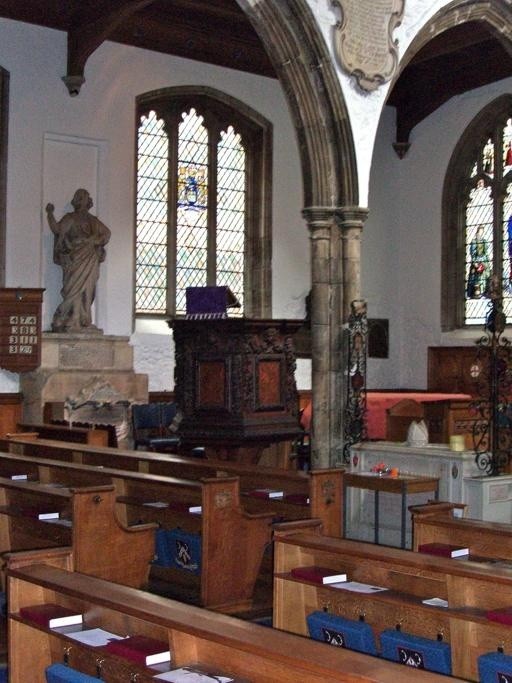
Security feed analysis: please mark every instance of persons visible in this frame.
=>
[467,223,494,299]
[42,189,110,331]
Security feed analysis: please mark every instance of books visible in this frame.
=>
[16,603,83,631]
[290,564,347,587]
[486,602,512,626]
[415,540,468,560]
[12,503,59,525]
[167,500,202,514]
[282,494,313,506]
[0,468,34,482]
[244,487,288,498]
[102,635,170,667]
